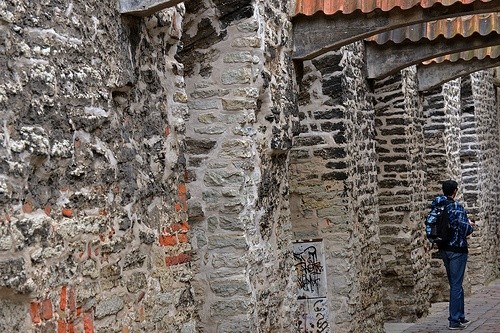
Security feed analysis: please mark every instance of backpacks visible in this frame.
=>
[425,200,456,247]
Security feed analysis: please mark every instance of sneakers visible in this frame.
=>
[459,319,471,325]
[448,324,466,329]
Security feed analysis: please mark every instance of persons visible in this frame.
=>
[430,180,476,332]
